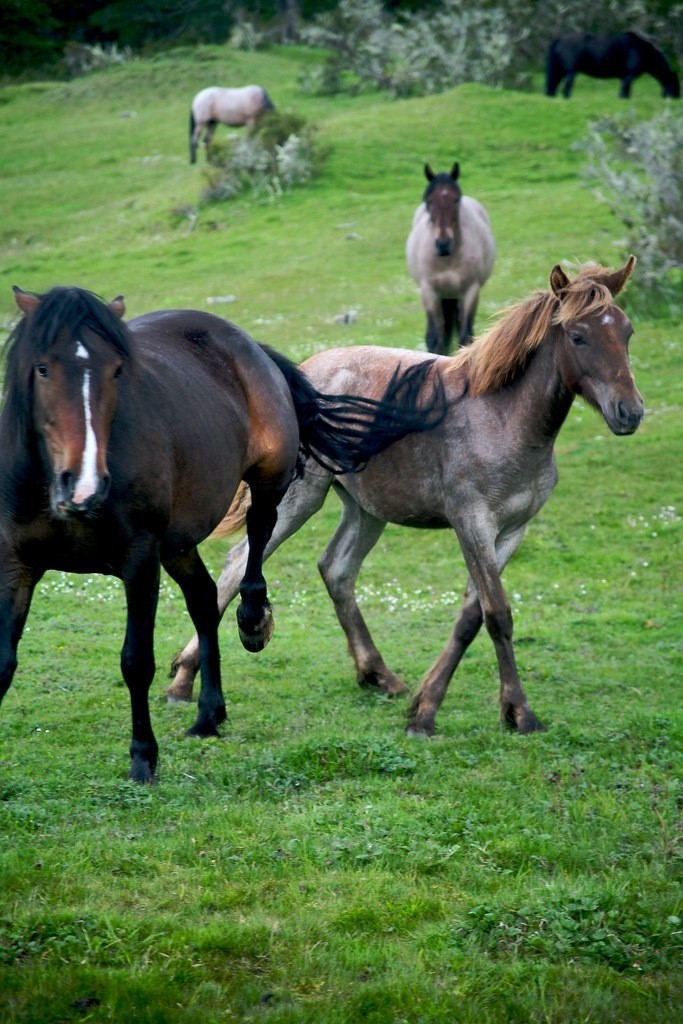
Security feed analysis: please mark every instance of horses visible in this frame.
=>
[187,82,281,166]
[0,283,473,789]
[404,161,497,356]
[168,254,645,737]
[541,25,683,102]
